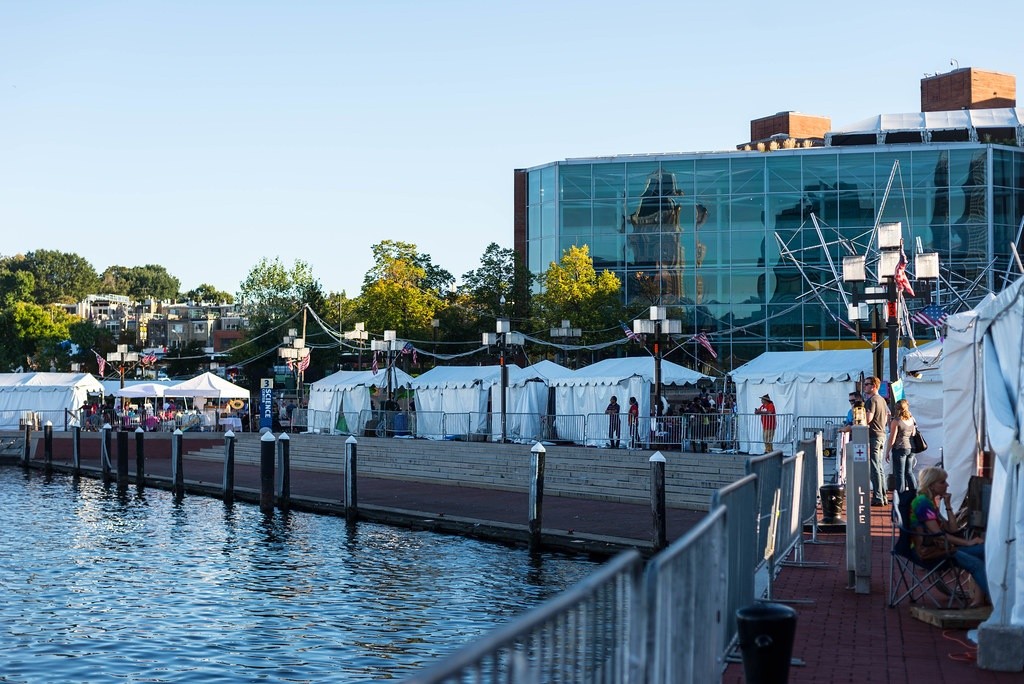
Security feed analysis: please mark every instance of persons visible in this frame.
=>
[911,466,990,608]
[838,377,890,507]
[652,368,737,448]
[605,396,622,449]
[627,396,642,450]
[754,394,778,454]
[80,398,300,433]
[886,399,920,489]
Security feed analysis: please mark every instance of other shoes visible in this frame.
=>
[871,499,888,507]
[638,448,642,450]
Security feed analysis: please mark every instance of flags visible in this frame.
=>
[372,348,380,375]
[96,352,105,377]
[142,353,156,364]
[401,341,417,362]
[620,320,636,342]
[298,356,310,373]
[692,332,719,358]
[911,302,949,332]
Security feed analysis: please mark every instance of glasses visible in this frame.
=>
[849,399,857,402]
[865,383,872,386]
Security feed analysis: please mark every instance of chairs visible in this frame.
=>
[890,489,968,611]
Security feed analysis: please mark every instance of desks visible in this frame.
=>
[219,418,242,433]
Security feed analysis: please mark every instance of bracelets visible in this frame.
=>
[945,508,953,511]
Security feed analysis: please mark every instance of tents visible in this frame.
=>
[117,383,170,430]
[163,372,252,434]
[729,346,909,454]
[0,373,105,430]
[552,357,716,446]
[906,277,1023,644]
[413,364,573,442]
[307,369,412,435]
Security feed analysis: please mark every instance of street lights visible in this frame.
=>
[171,329,182,353]
[633,306,682,419]
[369,329,406,401]
[278,338,309,405]
[481,318,526,445]
[106,344,139,429]
[841,220,939,424]
[550,319,581,370]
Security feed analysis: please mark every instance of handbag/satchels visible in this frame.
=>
[910,424,928,453]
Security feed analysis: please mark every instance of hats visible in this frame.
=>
[759,394,770,400]
